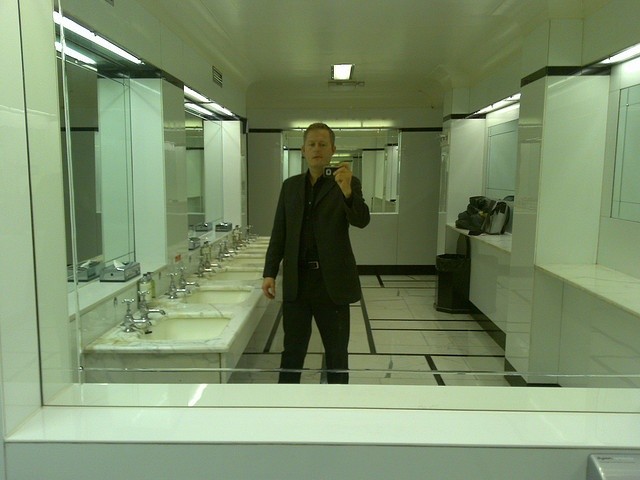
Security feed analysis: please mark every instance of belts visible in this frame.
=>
[297,259,321,271]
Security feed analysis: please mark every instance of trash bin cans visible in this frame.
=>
[433,253,473,314]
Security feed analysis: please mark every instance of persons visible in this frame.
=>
[262,123,371,383]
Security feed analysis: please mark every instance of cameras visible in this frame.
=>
[323,165,339,178]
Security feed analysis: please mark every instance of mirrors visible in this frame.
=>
[283,129,399,213]
[484,119,519,208]
[609,83,640,224]
[55,37,137,292]
[184,96,224,240]
[15,0,640,415]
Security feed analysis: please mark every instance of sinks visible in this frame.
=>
[205,269,270,280]
[138,312,232,344]
[182,285,253,305]
[226,254,276,263]
[237,244,270,251]
[248,236,274,243]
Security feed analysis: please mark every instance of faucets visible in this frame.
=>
[217,241,233,260]
[233,228,245,250]
[167,272,191,299]
[122,296,152,331]
[177,267,200,292]
[235,226,251,246]
[196,256,216,276]
[203,254,221,268]
[222,240,239,257]
[246,225,259,243]
[137,290,165,319]
